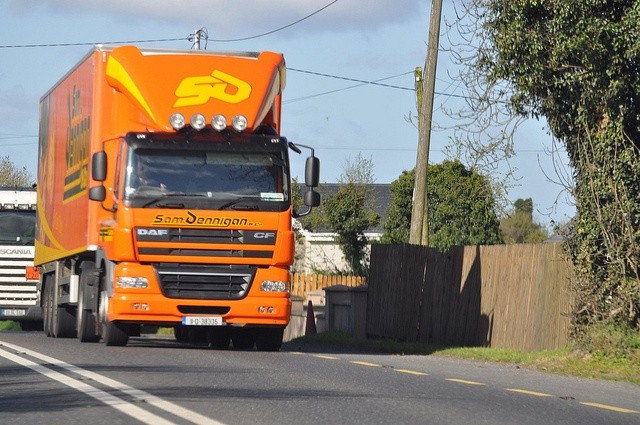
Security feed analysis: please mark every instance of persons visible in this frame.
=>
[134,170,168,190]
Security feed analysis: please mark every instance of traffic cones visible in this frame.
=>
[305,299,317,336]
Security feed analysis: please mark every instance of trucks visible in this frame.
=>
[38,45,322,350]
[1,186,41,330]
